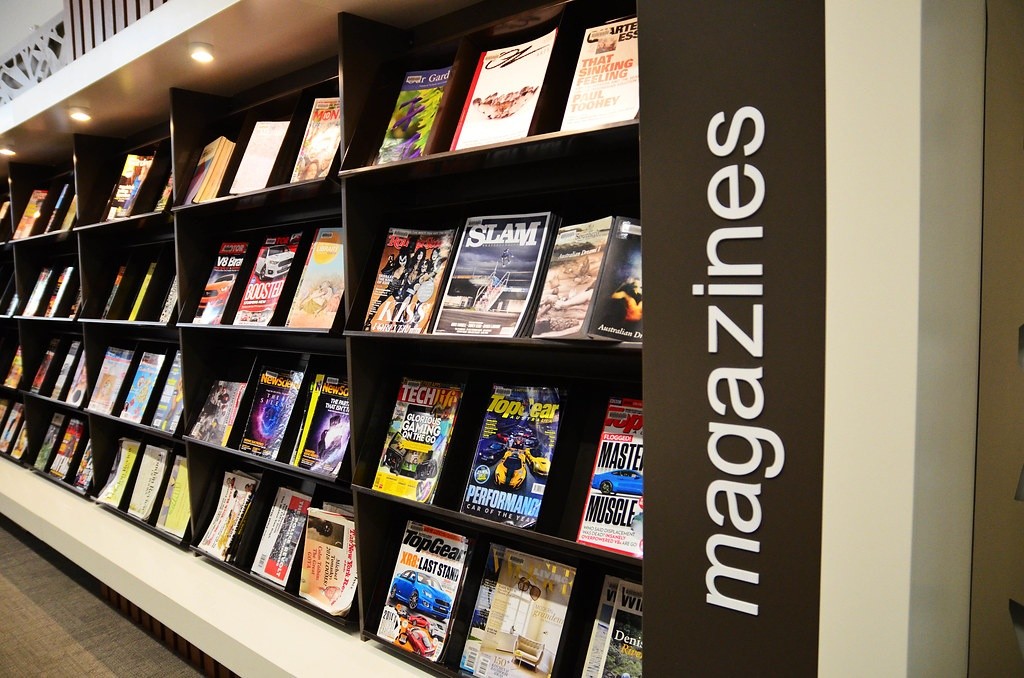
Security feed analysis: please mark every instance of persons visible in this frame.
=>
[4,404,24,441]
[501,248,514,267]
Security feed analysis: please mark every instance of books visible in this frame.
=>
[0,12,644,678]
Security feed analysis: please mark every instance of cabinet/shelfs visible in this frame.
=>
[0,0,644,678]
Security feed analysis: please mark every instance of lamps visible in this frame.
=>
[0,144,17,156]
[68,107,91,120]
[189,43,215,62]
